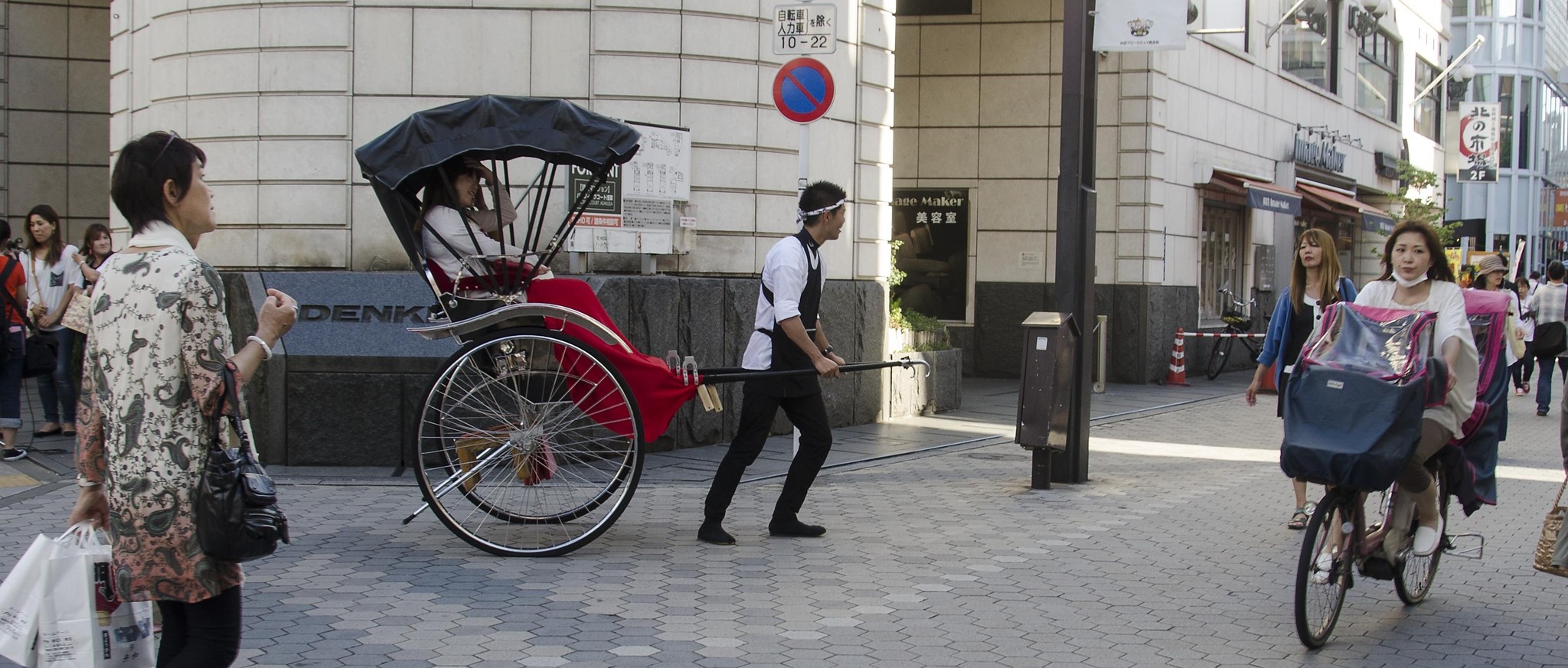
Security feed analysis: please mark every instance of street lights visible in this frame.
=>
[1442,56,1475,244]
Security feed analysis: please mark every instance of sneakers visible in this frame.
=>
[1522,381,1530,394]
[696,524,736,544]
[0,432,6,446]
[769,512,827,537]
[1515,388,1524,395]
[2,447,26,461]
[1537,408,1550,416]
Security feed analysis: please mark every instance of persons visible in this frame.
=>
[419,158,634,363]
[72,222,118,298]
[18,204,84,440]
[1313,220,1481,585]
[0,219,29,461]
[1467,254,1568,488]
[1245,228,1359,531]
[695,180,847,545]
[68,130,301,668]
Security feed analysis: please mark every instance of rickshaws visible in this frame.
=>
[344,89,935,559]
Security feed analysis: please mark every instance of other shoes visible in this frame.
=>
[64,428,77,436]
[34,426,61,436]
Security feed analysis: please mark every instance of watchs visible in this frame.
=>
[77,474,105,487]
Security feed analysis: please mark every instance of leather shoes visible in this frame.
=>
[1412,511,1444,556]
[1309,545,1343,584]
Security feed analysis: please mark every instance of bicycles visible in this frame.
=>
[1281,289,1512,654]
[1206,287,1278,380]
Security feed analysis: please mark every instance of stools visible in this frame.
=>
[456,422,532,492]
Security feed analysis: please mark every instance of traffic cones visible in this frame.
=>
[1163,327,1191,387]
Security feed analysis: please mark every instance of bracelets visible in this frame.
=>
[30,303,35,311]
[78,260,85,268]
[821,345,833,357]
[246,336,273,361]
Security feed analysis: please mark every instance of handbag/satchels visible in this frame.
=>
[1529,284,1568,357]
[61,289,92,335]
[0,525,81,667]
[37,528,160,668]
[1533,472,1568,578]
[21,331,59,378]
[192,362,291,564]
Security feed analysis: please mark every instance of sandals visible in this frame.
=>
[1287,508,1310,529]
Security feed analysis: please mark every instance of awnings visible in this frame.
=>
[1294,181,1396,235]
[1208,167,1303,218]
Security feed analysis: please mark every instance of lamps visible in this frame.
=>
[1347,0,1389,39]
[1296,122,1363,151]
[1300,0,1328,38]
[1446,62,1477,111]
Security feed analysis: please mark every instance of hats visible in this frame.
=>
[1476,255,1509,277]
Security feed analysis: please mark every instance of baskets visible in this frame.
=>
[1220,301,1256,332]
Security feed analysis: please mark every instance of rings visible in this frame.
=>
[293,305,299,312]
